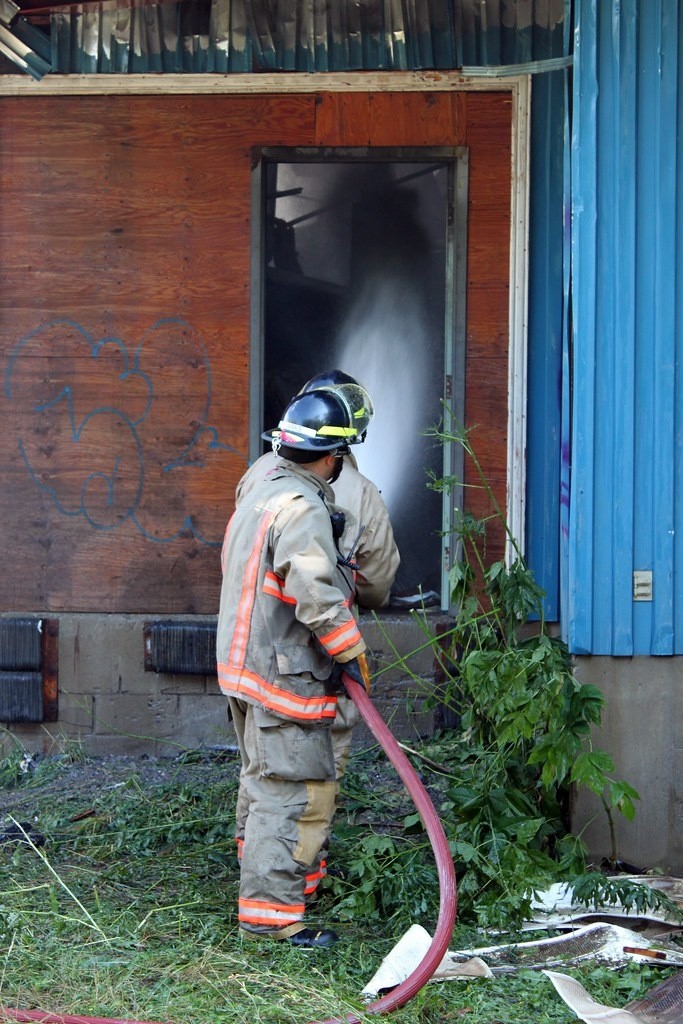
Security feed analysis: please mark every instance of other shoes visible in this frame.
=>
[305,890,319,905]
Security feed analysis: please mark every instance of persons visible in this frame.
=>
[216,383,375,948]
[235,369,400,905]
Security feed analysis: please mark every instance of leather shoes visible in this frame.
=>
[277,929,340,949]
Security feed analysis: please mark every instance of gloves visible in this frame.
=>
[340,654,369,701]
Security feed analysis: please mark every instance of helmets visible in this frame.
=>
[297,367,374,444]
[263,389,352,484]
